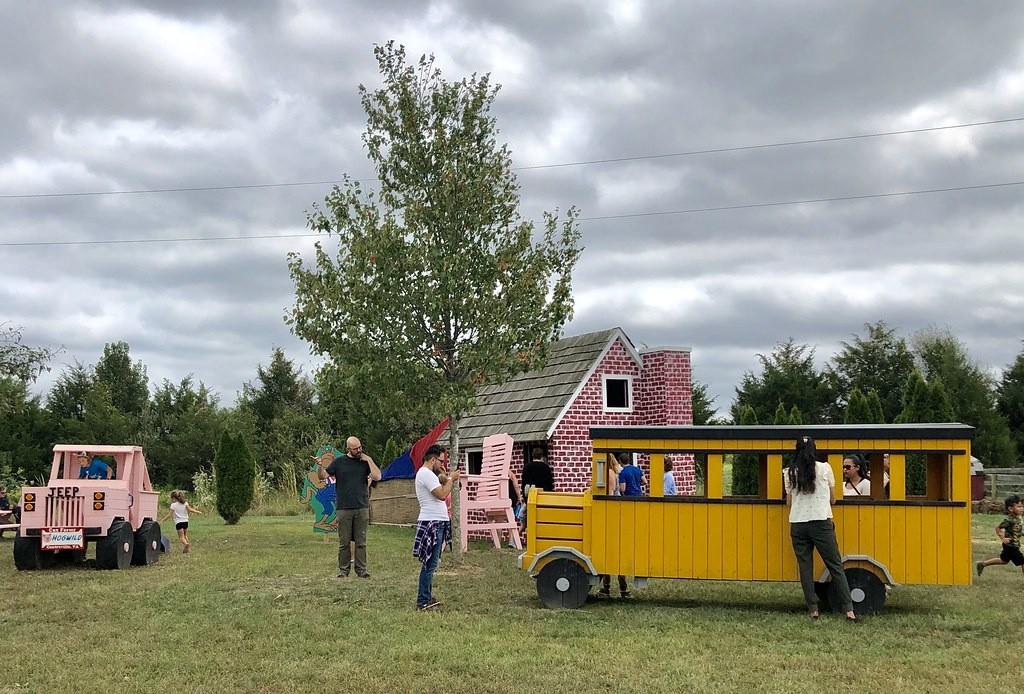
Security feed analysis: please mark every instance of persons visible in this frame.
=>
[444,494,452,553]
[844,453,871,496]
[310,437,381,579]
[664,457,677,497]
[414,445,460,612]
[159,492,202,554]
[867,454,890,488]
[977,495,1024,576]
[0,486,21,525]
[782,436,860,623]
[506,449,552,550]
[598,452,647,598]
[76,451,116,481]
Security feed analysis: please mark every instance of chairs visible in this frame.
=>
[459,433,523,551]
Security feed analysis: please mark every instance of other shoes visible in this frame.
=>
[183,543,190,554]
[338,571,349,577]
[597,589,611,595]
[416,598,441,611]
[810,615,818,621]
[359,573,370,578]
[619,591,632,597]
[508,540,517,548]
[976,561,984,576]
[846,616,864,623]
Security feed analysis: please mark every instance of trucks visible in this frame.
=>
[13,445,170,571]
[516,423,976,617]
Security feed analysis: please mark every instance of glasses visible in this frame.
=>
[884,455,890,459]
[843,465,855,470]
[1,492,7,494]
[435,457,444,464]
